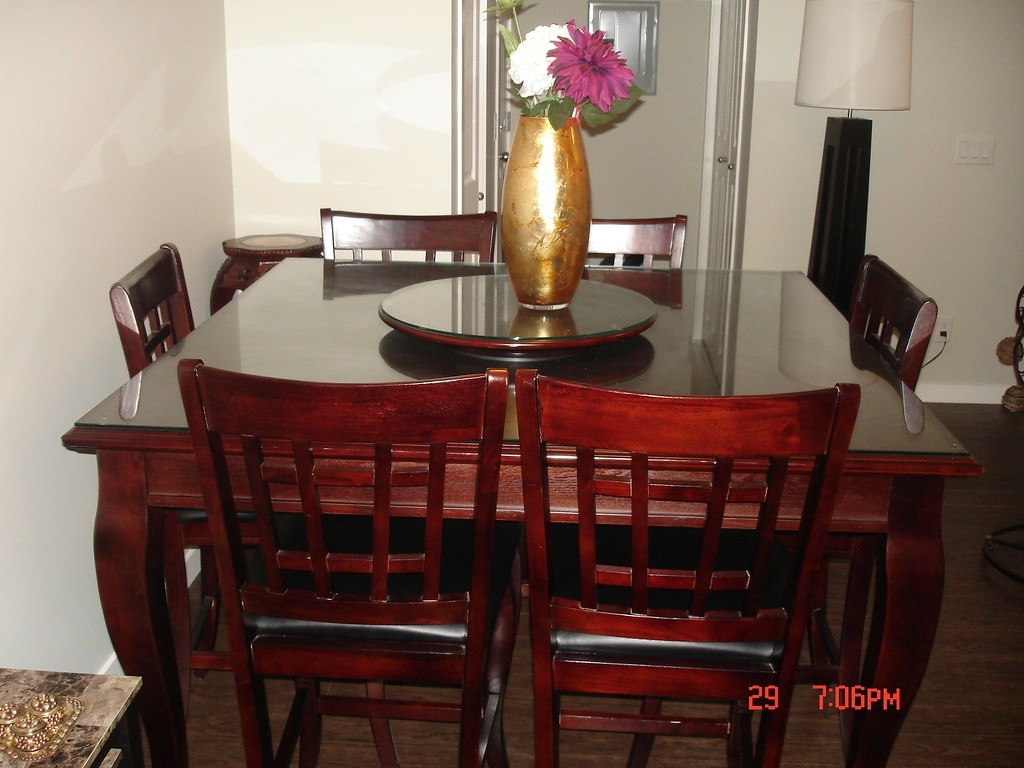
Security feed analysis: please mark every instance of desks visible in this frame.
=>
[60,257,989,768]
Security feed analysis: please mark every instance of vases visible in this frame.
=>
[502,116,592,311]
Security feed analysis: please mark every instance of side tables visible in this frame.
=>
[209,234,324,316]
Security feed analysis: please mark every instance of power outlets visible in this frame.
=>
[934,317,953,343]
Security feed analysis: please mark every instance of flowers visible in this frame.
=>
[487,0,648,131]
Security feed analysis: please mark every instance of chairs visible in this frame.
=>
[109,209,938,768]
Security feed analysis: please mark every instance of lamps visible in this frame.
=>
[794,0,914,322]
